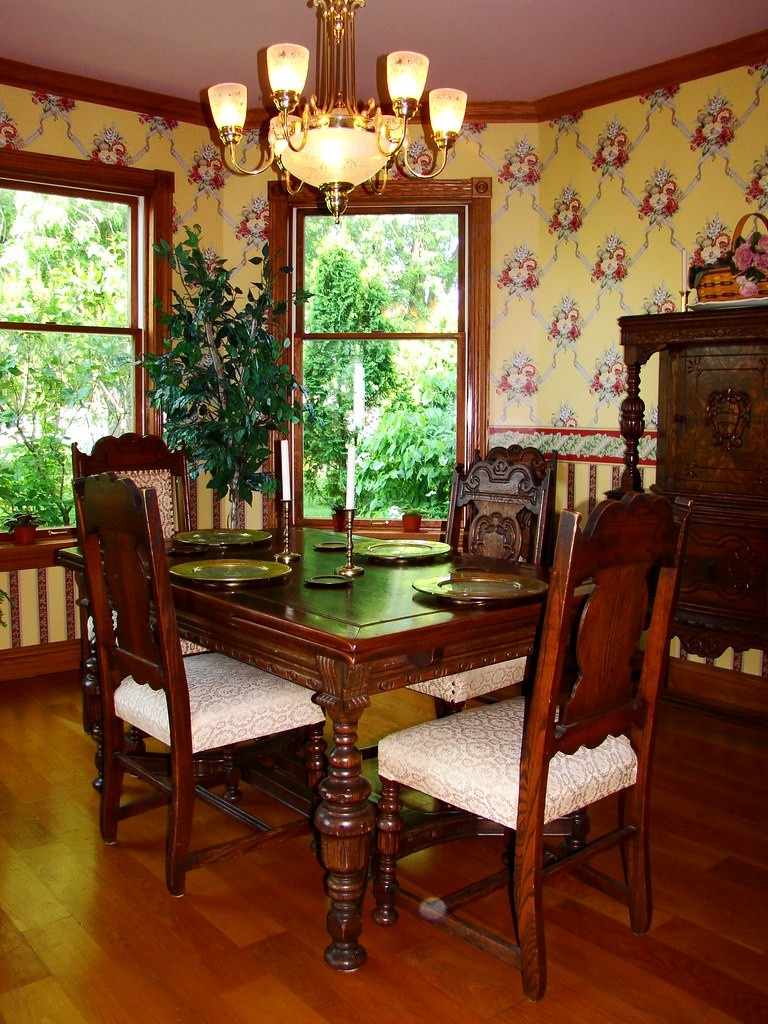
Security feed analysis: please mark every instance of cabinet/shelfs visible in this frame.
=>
[618,307,768,732]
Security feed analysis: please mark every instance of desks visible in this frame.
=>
[54,527,653,973]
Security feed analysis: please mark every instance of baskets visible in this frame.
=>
[697,213,768,302]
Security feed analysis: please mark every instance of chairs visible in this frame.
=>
[405,463,557,817]
[71,471,329,898]
[71,432,210,775]
[371,489,695,1003]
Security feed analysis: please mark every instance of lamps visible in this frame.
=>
[208,0,468,226]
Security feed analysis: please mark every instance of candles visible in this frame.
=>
[345,443,355,509]
[281,439,290,500]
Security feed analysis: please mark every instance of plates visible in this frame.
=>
[412,573,549,606]
[313,541,347,548]
[303,575,354,584]
[173,528,272,545]
[352,539,451,563]
[169,559,292,586]
[448,566,491,573]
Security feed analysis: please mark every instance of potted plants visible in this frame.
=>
[4,513,46,546]
[398,508,429,532]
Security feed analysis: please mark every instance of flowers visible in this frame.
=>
[689,232,768,298]
